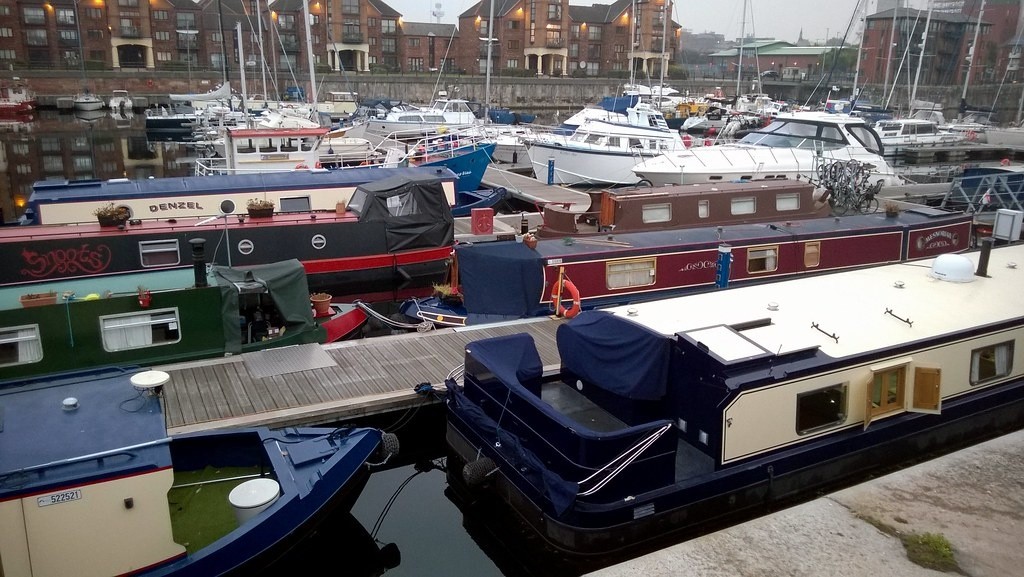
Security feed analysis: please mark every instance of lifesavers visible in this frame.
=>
[295,163,308,168]
[1000,159,1010,167]
[147,80,154,87]
[965,131,975,141]
[551,280,580,318]
[287,104,293,109]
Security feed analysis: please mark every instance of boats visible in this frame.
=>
[444,240,1024,570]
[0,0,1024,387]
[0,358,402,577]
